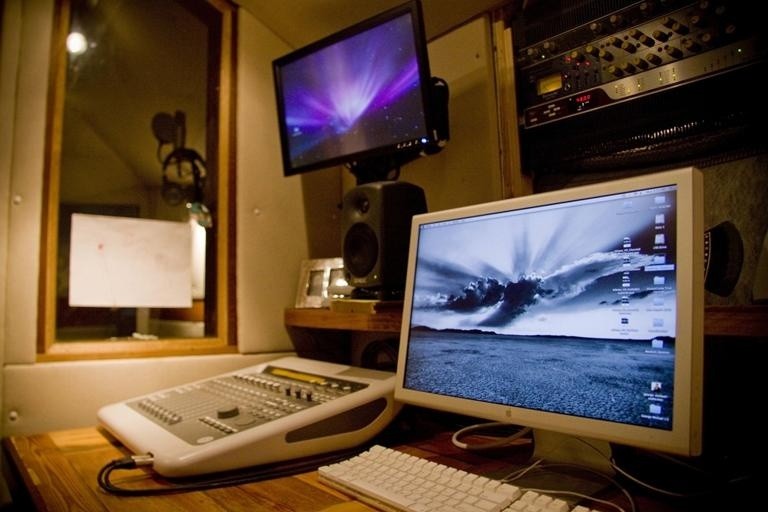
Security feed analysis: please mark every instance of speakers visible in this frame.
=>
[339,180,428,300]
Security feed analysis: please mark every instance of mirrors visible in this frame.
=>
[36,1,237,358]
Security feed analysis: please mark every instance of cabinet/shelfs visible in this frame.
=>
[5,299,768,511]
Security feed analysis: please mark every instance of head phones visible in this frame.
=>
[161,147,208,207]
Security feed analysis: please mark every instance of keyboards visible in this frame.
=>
[318,444,601,512]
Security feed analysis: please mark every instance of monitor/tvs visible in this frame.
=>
[272,0,449,186]
[392,166,705,506]
